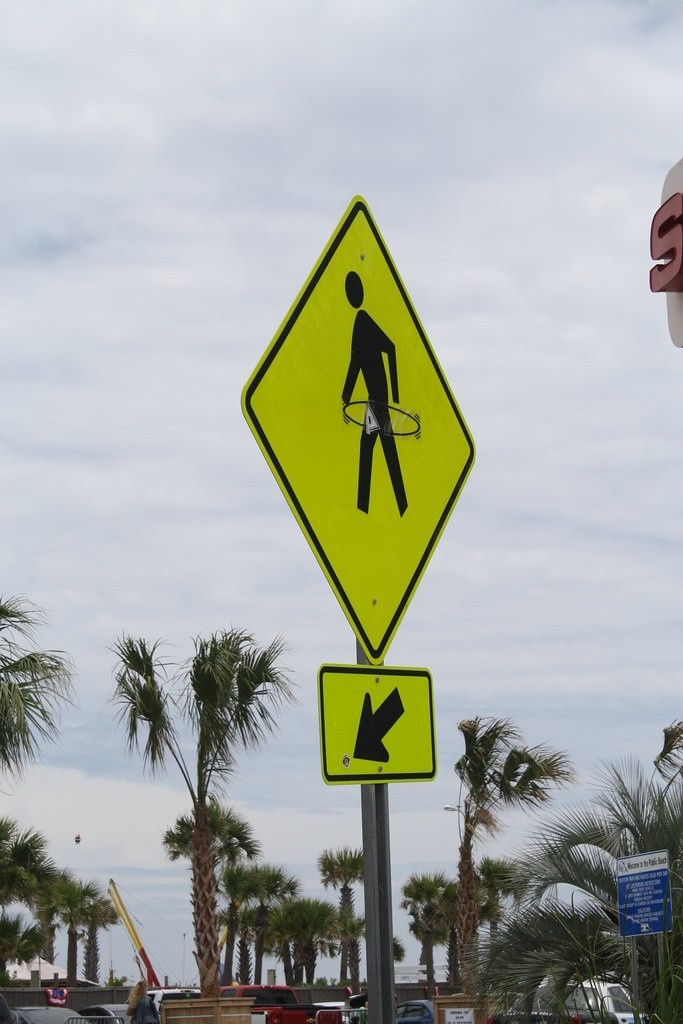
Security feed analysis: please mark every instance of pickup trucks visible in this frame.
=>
[220,983,344,1024]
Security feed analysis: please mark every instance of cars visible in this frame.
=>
[77,1004,136,1024]
[123,988,201,1014]
[9,1005,90,1024]
[395,1000,435,1024]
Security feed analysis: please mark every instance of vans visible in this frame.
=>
[529,974,646,1024]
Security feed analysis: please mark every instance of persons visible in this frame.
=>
[126,980,161,1024]
